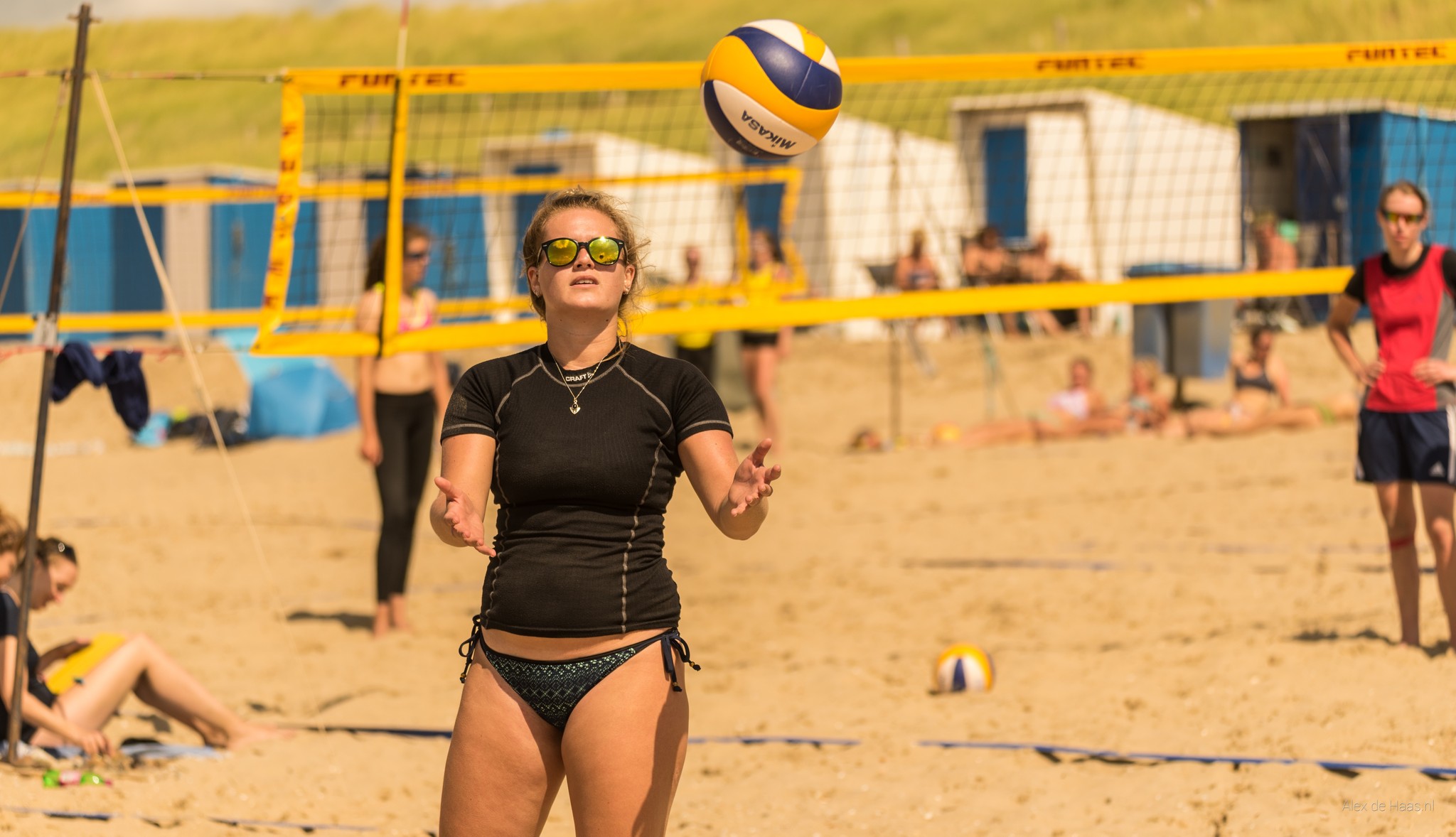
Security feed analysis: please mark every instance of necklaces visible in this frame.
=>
[554,356,606,415]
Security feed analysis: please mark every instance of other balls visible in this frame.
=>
[930,423,962,446]
[853,431,880,451]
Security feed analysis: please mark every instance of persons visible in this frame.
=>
[353,222,452,639]
[944,355,1173,445]
[649,223,795,454]
[1326,179,1456,647]
[1226,209,1299,419]
[962,224,1092,340]
[0,511,246,765]
[430,190,781,836]
[893,224,937,289]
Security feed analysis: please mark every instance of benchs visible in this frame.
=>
[956,226,1049,337]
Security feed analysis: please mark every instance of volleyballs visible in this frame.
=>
[701,19,843,160]
[933,641,996,692]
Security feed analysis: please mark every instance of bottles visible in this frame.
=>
[43,770,113,787]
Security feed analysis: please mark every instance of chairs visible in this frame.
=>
[860,258,911,332]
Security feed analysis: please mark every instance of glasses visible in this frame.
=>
[407,251,427,260]
[1382,210,1424,224]
[536,236,628,269]
